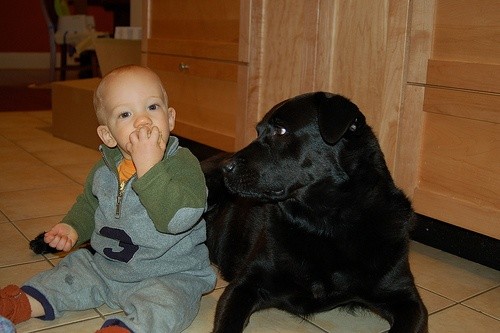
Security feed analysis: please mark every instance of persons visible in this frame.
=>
[0,64,217,333]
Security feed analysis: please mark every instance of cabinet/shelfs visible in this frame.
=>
[94,38,141,78]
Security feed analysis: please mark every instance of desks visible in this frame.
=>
[56,32,107,80]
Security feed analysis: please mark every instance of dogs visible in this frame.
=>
[29,91,428,333]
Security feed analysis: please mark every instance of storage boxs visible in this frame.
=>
[29,77,107,151]
